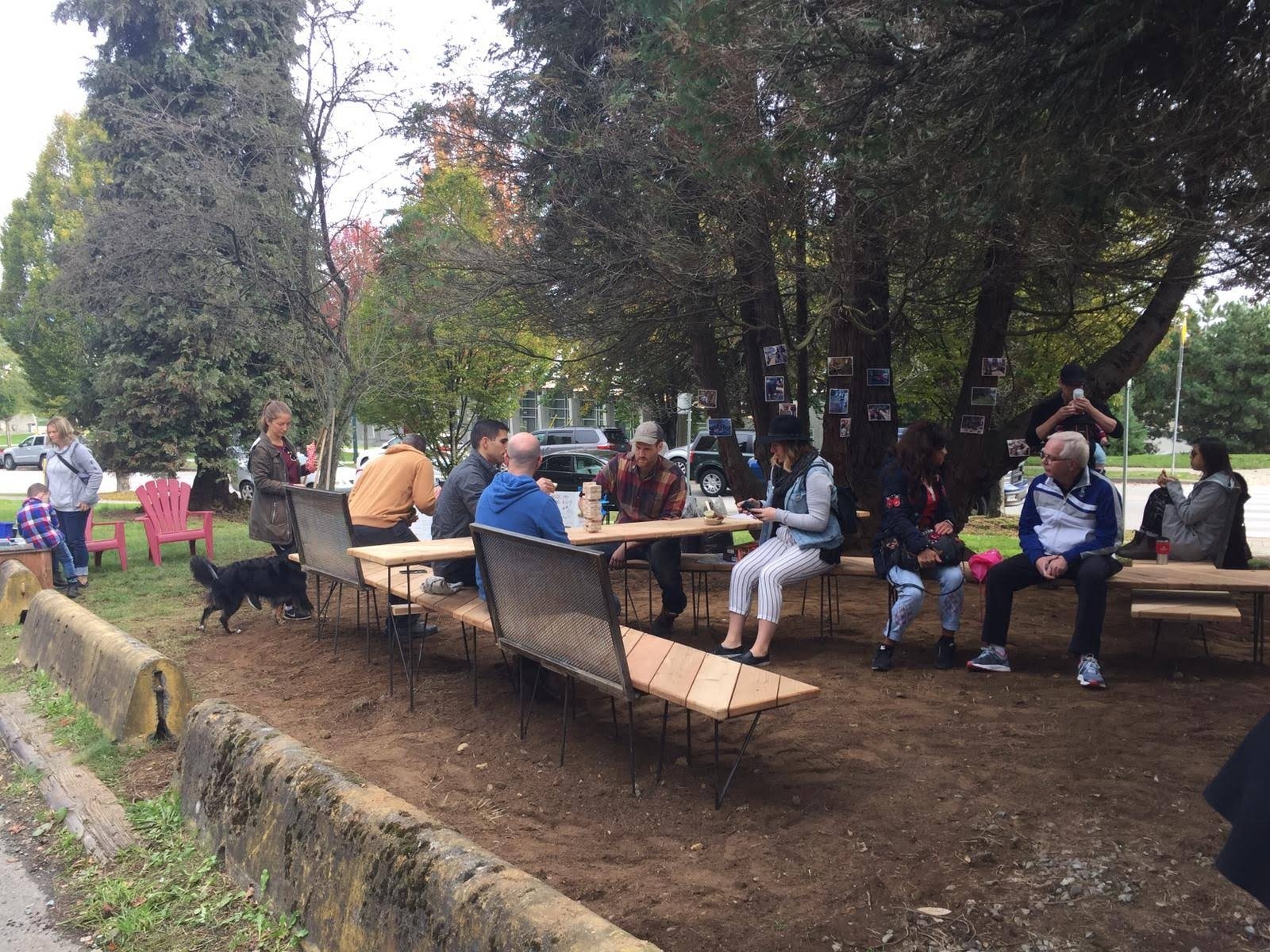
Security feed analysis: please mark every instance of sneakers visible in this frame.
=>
[872,641,894,671]
[966,645,1011,672]
[933,634,956,670]
[283,608,312,620]
[1077,652,1106,688]
[245,591,262,610]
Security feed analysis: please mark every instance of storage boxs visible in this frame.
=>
[0,522,15,538]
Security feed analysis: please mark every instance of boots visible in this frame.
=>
[1115,529,1159,559]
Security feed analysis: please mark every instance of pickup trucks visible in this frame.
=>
[3,434,52,470]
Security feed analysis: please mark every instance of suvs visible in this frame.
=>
[690,430,757,497]
[355,433,470,487]
[495,425,632,473]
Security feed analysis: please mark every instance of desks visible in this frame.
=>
[0,549,54,589]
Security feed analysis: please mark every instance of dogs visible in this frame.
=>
[189,552,313,634]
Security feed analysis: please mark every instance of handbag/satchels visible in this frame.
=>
[52,450,90,485]
[900,525,966,573]
[880,535,902,568]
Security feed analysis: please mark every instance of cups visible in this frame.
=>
[12,524,18,538]
[1073,389,1083,399]
[1156,537,1170,564]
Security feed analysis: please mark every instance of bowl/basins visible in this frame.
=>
[703,515,726,525]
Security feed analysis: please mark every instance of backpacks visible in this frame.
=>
[804,464,871,535]
[968,547,1004,625]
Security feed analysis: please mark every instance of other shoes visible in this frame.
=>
[65,579,90,589]
[519,654,562,698]
[52,578,66,589]
[710,642,745,657]
[731,648,771,667]
[67,581,82,597]
[652,606,678,636]
[385,620,438,638]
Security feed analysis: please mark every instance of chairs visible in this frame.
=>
[85,508,127,570]
[133,479,214,567]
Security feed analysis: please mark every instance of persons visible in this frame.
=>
[16,482,81,598]
[711,411,844,668]
[1025,364,1240,568]
[43,416,102,589]
[871,418,964,671]
[348,432,438,640]
[594,421,687,632]
[966,430,1124,688]
[249,400,319,620]
[430,419,622,699]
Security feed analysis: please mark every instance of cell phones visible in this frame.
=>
[740,500,757,515]
[1073,388,1084,400]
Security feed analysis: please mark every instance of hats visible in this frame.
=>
[629,421,663,445]
[760,415,814,443]
[1061,363,1086,382]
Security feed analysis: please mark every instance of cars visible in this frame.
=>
[533,449,619,491]
[230,451,358,501]
[663,442,693,476]
[1002,460,1030,508]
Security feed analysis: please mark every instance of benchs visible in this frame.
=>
[280,481,1270,810]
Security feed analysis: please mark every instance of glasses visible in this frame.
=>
[1191,451,1200,458]
[1062,381,1085,390]
